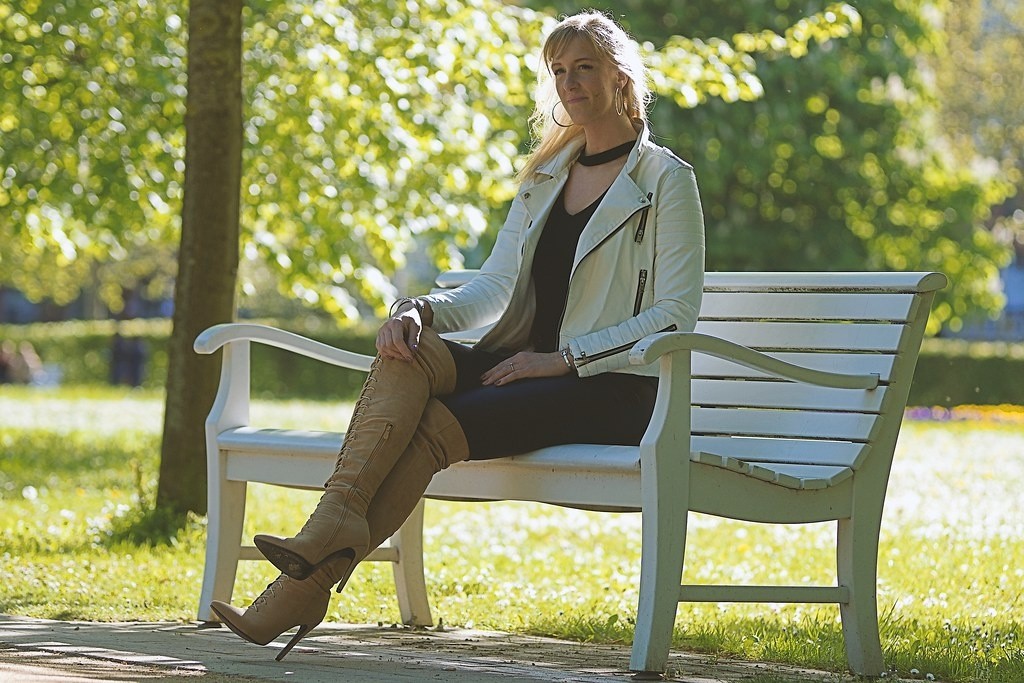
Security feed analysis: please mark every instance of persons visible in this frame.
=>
[209,13,707,662]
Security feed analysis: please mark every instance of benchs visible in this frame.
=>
[185,269,949,679]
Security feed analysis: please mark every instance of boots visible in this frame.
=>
[208,325,471,653]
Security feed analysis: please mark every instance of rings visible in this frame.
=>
[509,363,515,372]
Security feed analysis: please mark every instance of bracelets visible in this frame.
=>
[388,296,426,318]
[561,349,575,372]
